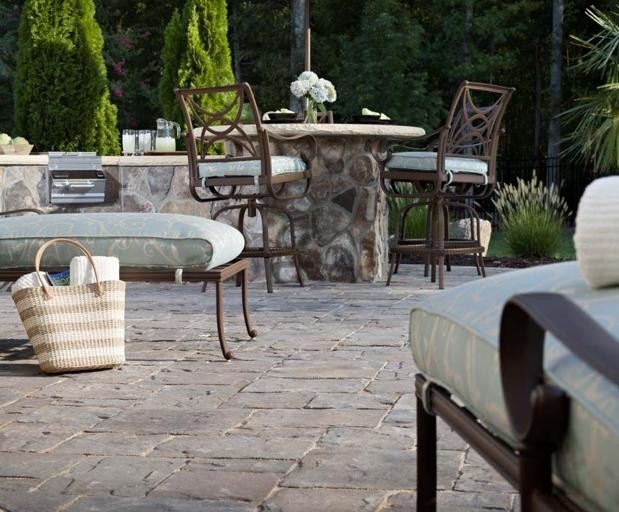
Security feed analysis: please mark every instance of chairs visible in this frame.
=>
[408,175,618,511]
[178,83,318,292]
[378,83,515,291]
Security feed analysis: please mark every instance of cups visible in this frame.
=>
[121,128,155,156]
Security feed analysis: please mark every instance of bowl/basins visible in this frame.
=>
[351,114,381,121]
[1,143,34,156]
[267,111,300,120]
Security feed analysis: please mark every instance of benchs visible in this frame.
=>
[1,209,255,366]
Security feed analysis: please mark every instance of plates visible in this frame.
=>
[261,120,306,124]
[345,120,399,125]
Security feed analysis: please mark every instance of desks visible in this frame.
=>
[194,124,425,283]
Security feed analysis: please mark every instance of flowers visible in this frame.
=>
[290,71,337,124]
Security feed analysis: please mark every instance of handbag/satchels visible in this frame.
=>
[11,237,129,376]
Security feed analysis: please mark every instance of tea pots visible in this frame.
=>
[155,117,181,152]
